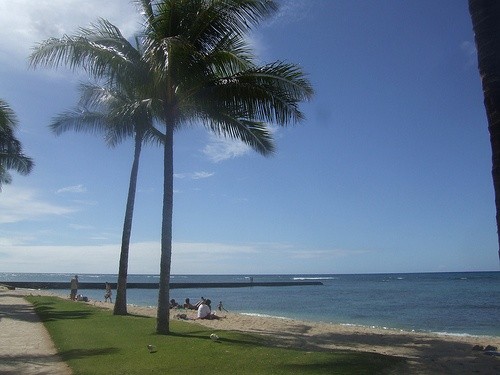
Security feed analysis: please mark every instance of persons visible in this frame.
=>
[183,298,197,309]
[168,299,182,309]
[70,275,78,301]
[104,282,112,303]
[198,299,226,319]
[218,301,223,311]
